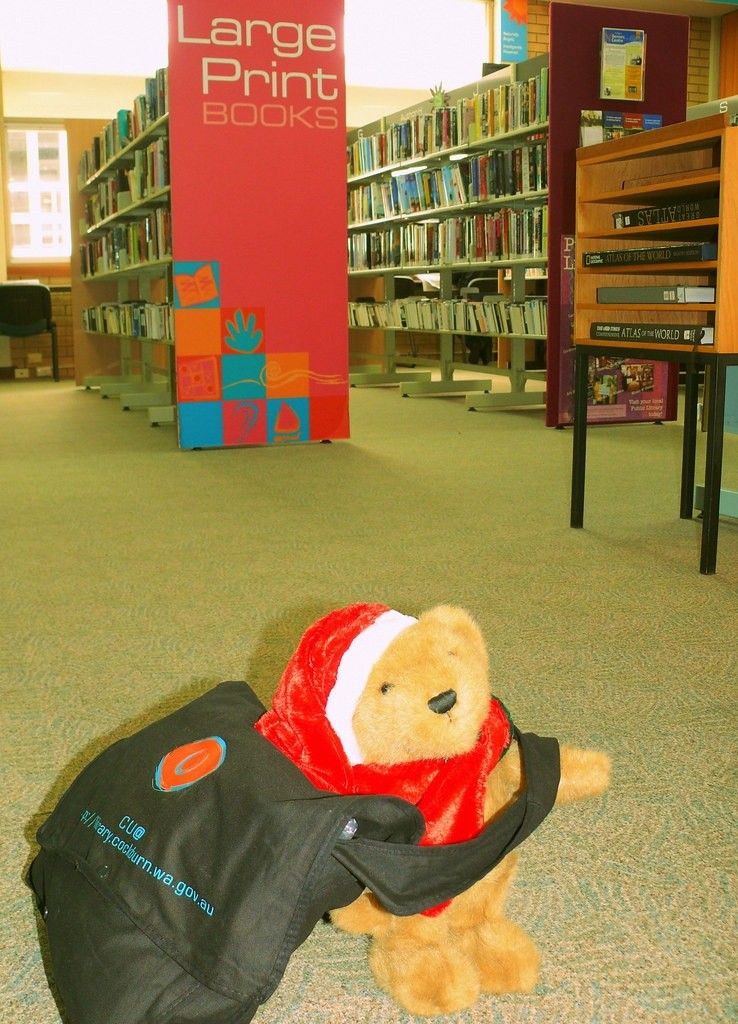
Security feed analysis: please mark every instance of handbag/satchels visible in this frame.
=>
[24,683,561,1024]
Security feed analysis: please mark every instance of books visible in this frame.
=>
[589,321,714,346]
[77,67,174,341]
[597,284,715,304]
[582,242,719,267]
[613,199,719,229]
[348,66,550,336]
[580,109,661,148]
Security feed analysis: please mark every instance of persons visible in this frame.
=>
[587,353,654,406]
[452,269,506,365]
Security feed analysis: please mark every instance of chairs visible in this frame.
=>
[0,282,61,384]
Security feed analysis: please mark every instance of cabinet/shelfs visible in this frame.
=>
[73,0,738,579]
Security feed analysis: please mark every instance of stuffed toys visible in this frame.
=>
[253,602,613,1017]
